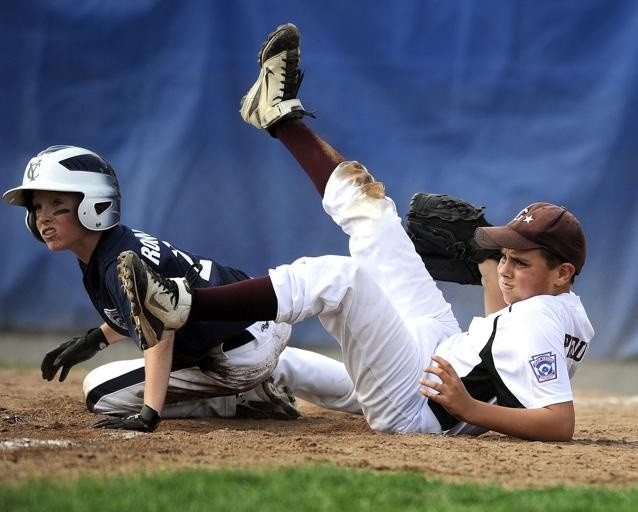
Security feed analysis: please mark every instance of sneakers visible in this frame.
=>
[241,24,305,136]
[238,375,301,419]
[117,251,192,350]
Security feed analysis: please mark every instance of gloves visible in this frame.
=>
[89,405,161,432]
[41,328,109,382]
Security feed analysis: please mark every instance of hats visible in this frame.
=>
[475,202,585,275]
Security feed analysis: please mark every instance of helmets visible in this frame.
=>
[2,145,120,243]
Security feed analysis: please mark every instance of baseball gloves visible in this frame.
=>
[406,193,501,285]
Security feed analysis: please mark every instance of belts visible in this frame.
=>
[218,329,255,352]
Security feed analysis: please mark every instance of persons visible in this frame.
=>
[117,22,599,445]
[3,144,303,433]
